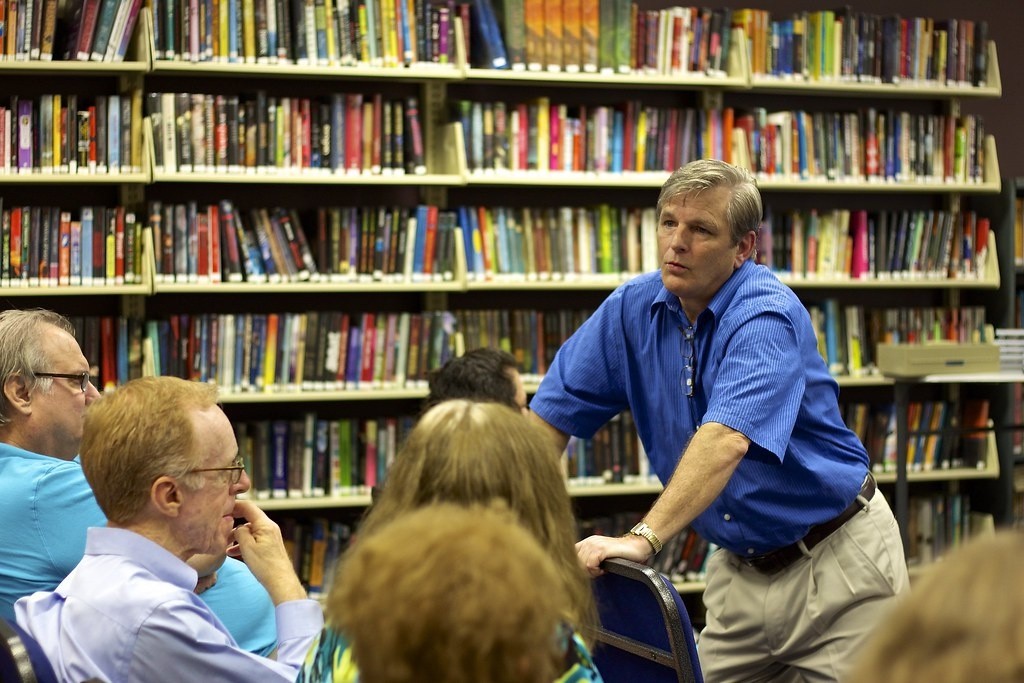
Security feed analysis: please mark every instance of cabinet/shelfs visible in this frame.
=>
[0,0,1024,683]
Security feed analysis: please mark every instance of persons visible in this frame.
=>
[0,310,325,683]
[297,346,603,683]
[528,159,912,683]
[844,529,1024,683]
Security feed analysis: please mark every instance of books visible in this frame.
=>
[0,0,989,596]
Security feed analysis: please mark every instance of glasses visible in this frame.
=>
[678,326,695,398]
[152,456,246,485]
[29,370,90,392]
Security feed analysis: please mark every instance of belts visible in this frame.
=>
[736,472,876,575]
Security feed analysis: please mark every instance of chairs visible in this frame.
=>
[583,558,705,683]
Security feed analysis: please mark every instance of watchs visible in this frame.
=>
[630,522,663,555]
